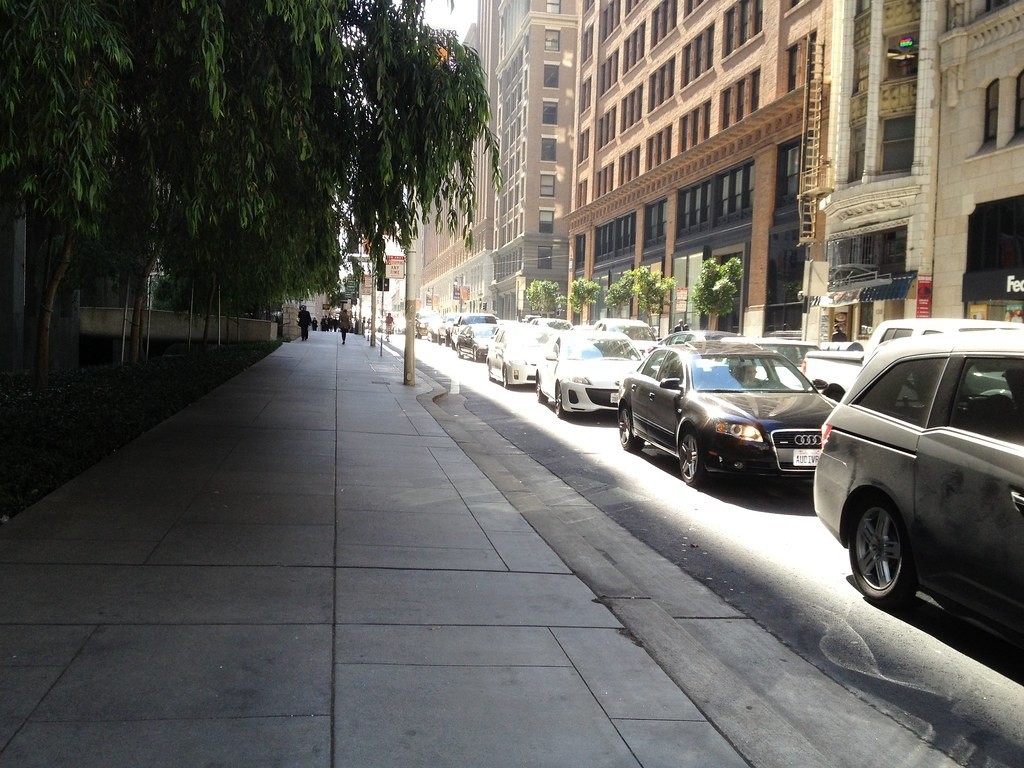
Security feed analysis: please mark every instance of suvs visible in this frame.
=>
[811,327,1024,652]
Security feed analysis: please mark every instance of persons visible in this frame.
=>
[738,362,764,388]
[684,324,690,331]
[298,306,311,341]
[312,317,317,331]
[832,324,848,342]
[385,312,393,336]
[338,309,350,345]
[674,319,686,333]
[320,314,339,332]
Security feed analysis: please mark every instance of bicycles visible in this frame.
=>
[385,323,394,343]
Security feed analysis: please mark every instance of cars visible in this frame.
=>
[358,301,1024,393]
[618,340,835,488]
[457,322,496,364]
[485,324,560,390]
[535,329,645,419]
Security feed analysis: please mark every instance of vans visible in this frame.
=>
[860,318,1024,403]
